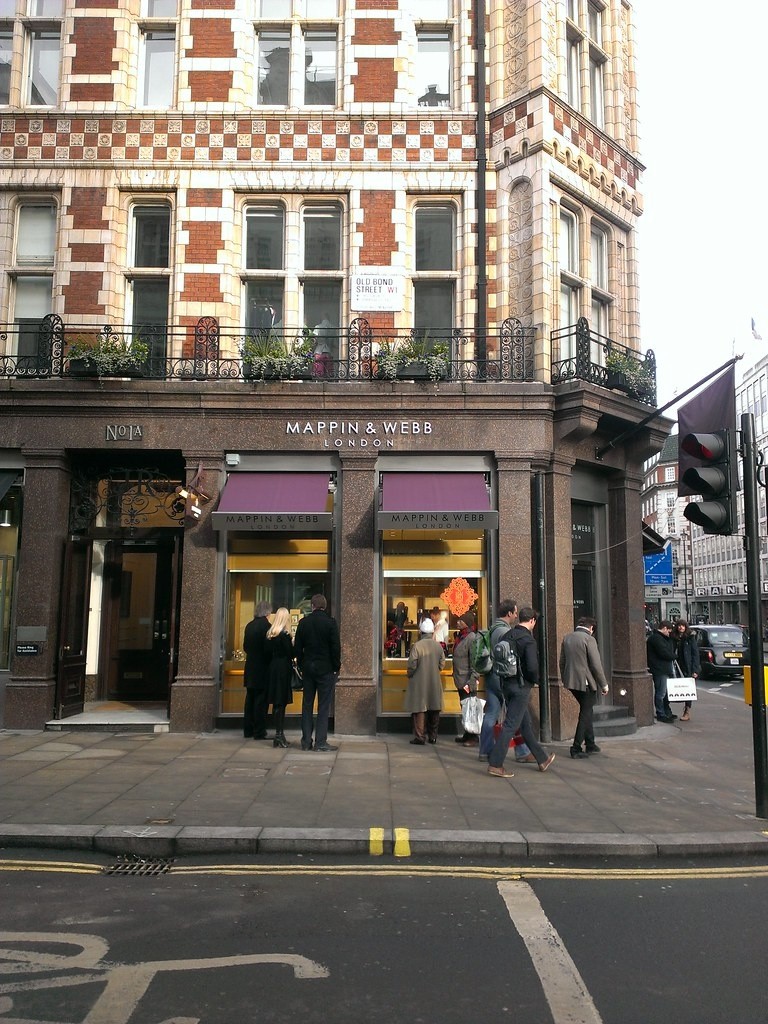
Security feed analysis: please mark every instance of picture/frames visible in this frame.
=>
[120,571,133,617]
[291,614,299,636]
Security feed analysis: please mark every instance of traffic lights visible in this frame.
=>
[681,428,738,535]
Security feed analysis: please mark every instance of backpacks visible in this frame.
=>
[494,631,531,677]
[472,623,510,674]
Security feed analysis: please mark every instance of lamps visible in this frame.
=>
[0,510,12,526]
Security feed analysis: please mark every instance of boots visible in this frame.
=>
[680,705,691,722]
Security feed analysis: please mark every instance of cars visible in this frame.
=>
[688,624,749,681]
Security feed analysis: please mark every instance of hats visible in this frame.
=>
[459,611,474,628]
[420,618,435,634]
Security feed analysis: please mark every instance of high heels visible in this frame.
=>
[273,728,292,747]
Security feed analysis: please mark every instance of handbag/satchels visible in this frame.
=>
[666,678,697,702]
[494,700,524,747]
[460,694,487,735]
[291,658,303,690]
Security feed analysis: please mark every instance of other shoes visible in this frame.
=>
[571,751,588,759]
[258,735,274,740]
[538,751,555,771]
[410,737,424,745]
[455,735,466,742]
[658,715,674,723]
[669,713,678,720]
[487,766,516,777]
[463,740,479,747]
[517,754,536,763]
[478,754,489,762]
[586,746,600,753]
[429,737,436,743]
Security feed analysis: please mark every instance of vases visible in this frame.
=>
[70,359,141,378]
[610,373,646,394]
[242,363,312,380]
[390,647,396,659]
[379,362,447,380]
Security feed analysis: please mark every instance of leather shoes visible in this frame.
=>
[302,744,311,751]
[314,742,339,752]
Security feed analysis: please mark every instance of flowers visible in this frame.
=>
[66,322,149,389]
[601,345,655,403]
[375,327,450,397]
[228,320,319,387]
[384,626,404,649]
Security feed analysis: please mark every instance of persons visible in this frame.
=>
[407,619,446,744]
[559,617,608,758]
[313,313,334,376]
[242,601,295,747]
[294,594,342,751]
[488,607,555,777]
[453,612,479,746]
[478,600,537,763]
[645,617,700,723]
[430,610,449,648]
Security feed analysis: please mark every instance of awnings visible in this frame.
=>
[642,520,666,555]
[210,471,334,531]
[377,471,499,529]
